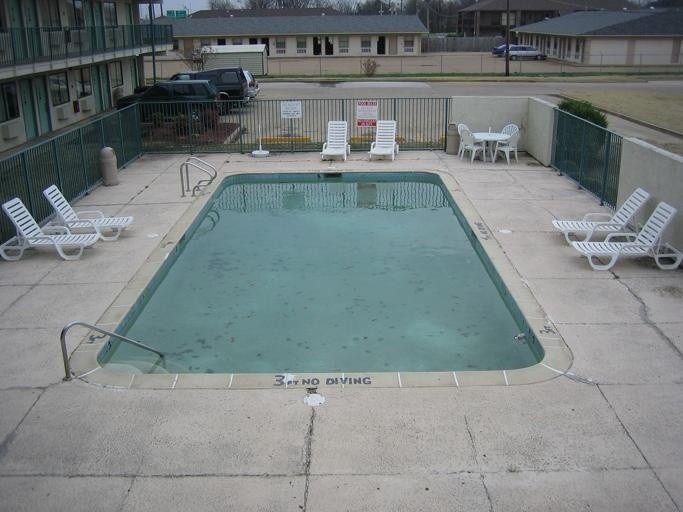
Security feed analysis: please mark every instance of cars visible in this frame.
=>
[491,44,547,61]
[116,66,261,123]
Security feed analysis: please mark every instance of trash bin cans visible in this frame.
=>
[446,123,460,155]
[98,147,119,186]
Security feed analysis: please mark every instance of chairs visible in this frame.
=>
[40,183,132,244]
[368,120,400,160]
[319,118,351,163]
[551,187,651,245]
[457,122,520,165]
[1,197,99,263]
[571,200,683,271]
